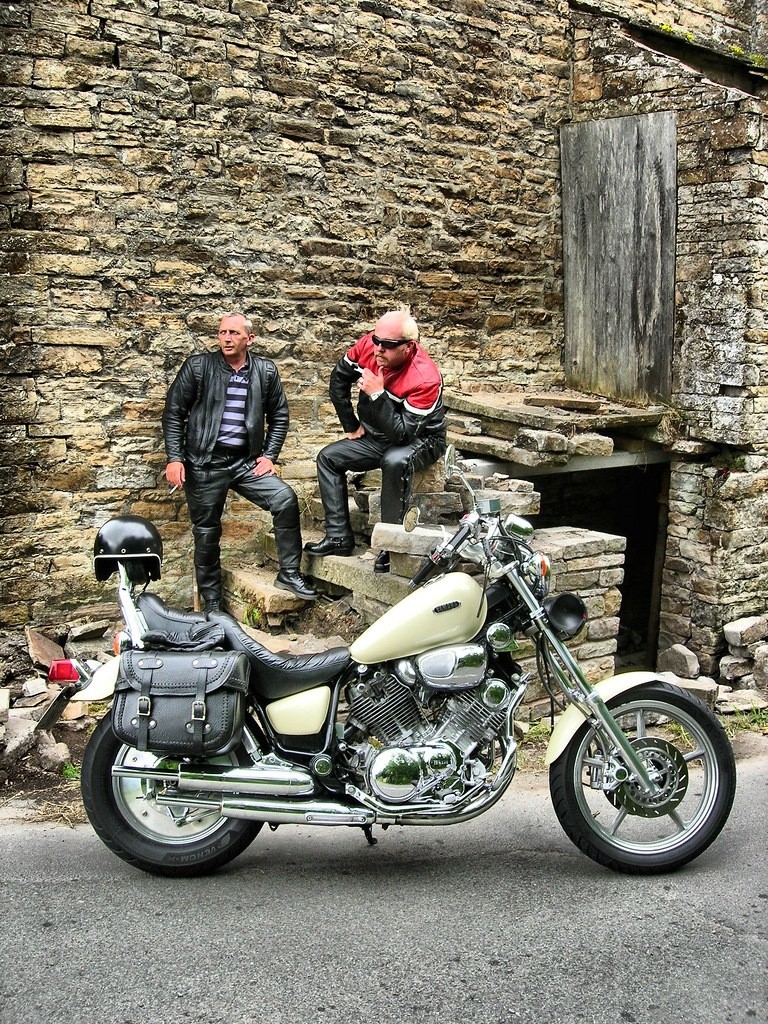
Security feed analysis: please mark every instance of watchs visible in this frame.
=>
[369,389,385,401]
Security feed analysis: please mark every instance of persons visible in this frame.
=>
[301,311,447,573]
[161,312,321,622]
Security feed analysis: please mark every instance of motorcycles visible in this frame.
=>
[25,447,734,875]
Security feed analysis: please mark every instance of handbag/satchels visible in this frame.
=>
[112,648,251,758]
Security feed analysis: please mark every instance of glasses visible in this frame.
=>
[372,334,412,350]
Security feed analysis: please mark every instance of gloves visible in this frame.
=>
[140,621,226,651]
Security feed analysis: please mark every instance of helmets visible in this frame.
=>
[94,515,163,585]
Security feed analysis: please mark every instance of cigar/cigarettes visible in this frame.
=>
[169,480,182,495]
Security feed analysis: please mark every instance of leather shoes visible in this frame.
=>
[303,535,355,556]
[274,568,320,600]
[205,600,220,620]
[373,550,390,573]
